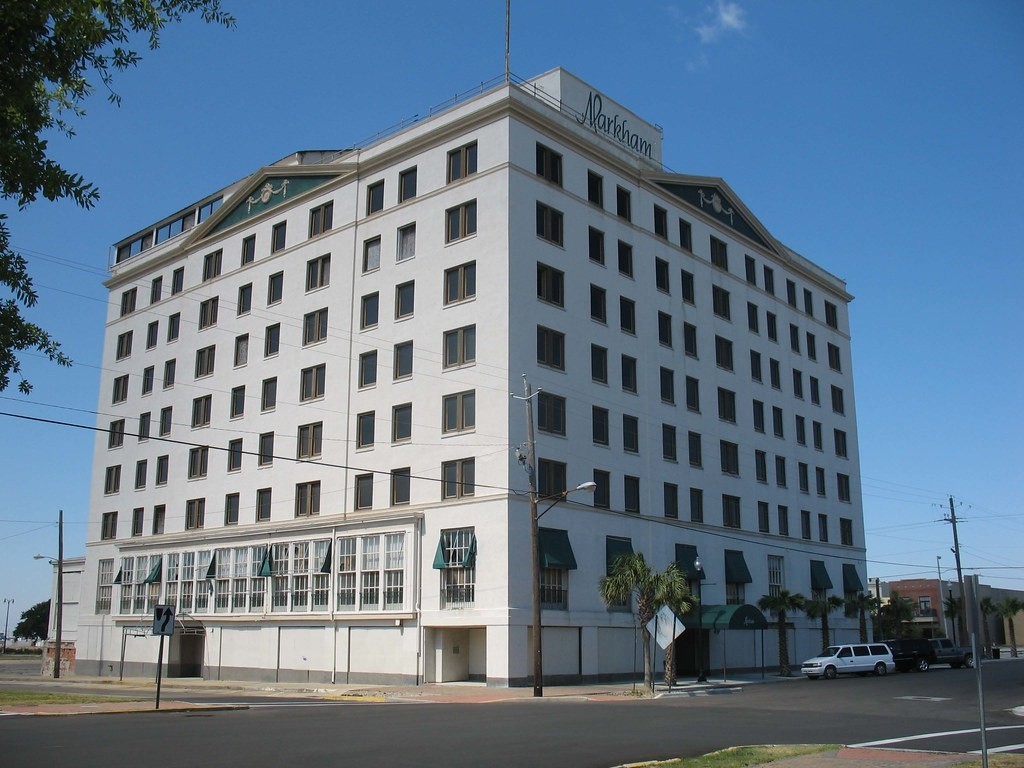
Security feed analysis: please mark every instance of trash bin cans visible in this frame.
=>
[991,647,1001,659]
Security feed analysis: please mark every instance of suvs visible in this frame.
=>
[879,636,939,672]
[801,643,896,680]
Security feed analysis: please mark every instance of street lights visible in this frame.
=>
[946,577,959,640]
[3,597,15,653]
[937,555,949,639]
[693,555,710,682]
[33,553,64,678]
[532,481,596,697]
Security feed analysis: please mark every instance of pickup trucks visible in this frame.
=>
[928,637,983,669]
